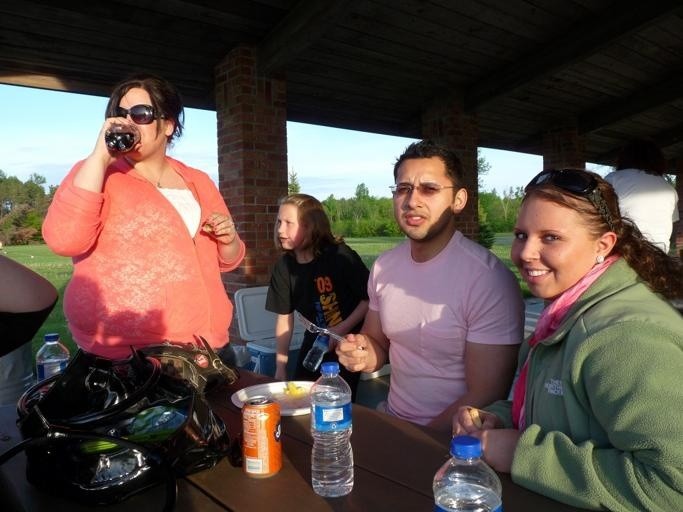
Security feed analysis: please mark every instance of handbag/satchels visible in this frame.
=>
[0,348,231,512]
[128,335,239,398]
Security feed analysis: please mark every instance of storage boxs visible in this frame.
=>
[235,285,305,381]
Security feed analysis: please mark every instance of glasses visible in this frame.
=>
[389,182,458,196]
[525,167,616,233]
[116,104,167,125]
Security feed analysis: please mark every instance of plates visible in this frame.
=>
[230,381,315,416]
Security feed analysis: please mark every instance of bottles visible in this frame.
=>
[35,334,70,384]
[310,362,355,499]
[302,331,330,372]
[431,436,502,512]
[104,125,140,152]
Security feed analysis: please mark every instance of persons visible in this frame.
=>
[334,139,526,434]
[42,70,246,360]
[265,195,368,401]
[0,256,58,356]
[449,165,683,512]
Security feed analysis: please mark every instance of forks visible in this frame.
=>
[293,311,363,350]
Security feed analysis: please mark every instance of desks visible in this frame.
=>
[0,366,590,512]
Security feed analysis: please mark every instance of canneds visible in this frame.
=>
[241,395,284,480]
